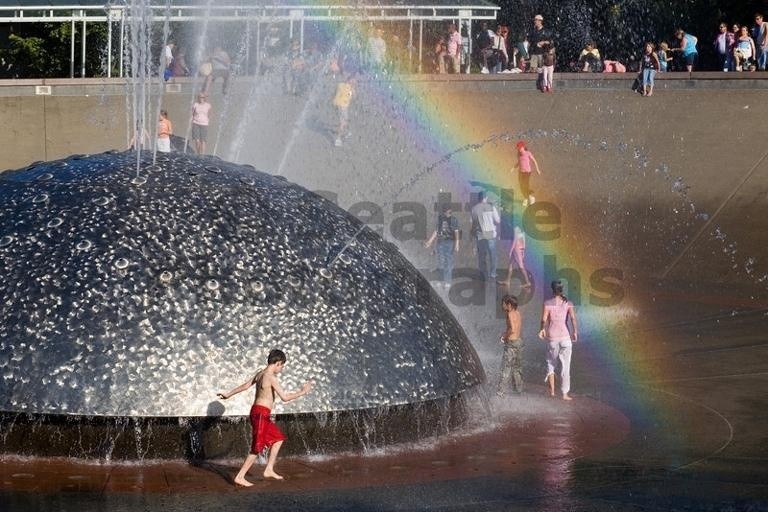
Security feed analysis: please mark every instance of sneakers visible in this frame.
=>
[542,86,552,93]
[529,196,536,205]
[643,91,653,97]
[522,199,529,206]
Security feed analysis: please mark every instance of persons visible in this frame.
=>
[215,347,313,488]
[509,141,541,207]
[128,26,358,159]
[422,202,461,289]
[466,190,502,281]
[362,25,387,75]
[535,280,577,401]
[425,12,767,97]
[494,294,526,397]
[494,212,532,289]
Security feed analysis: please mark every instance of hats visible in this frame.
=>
[515,141,525,150]
